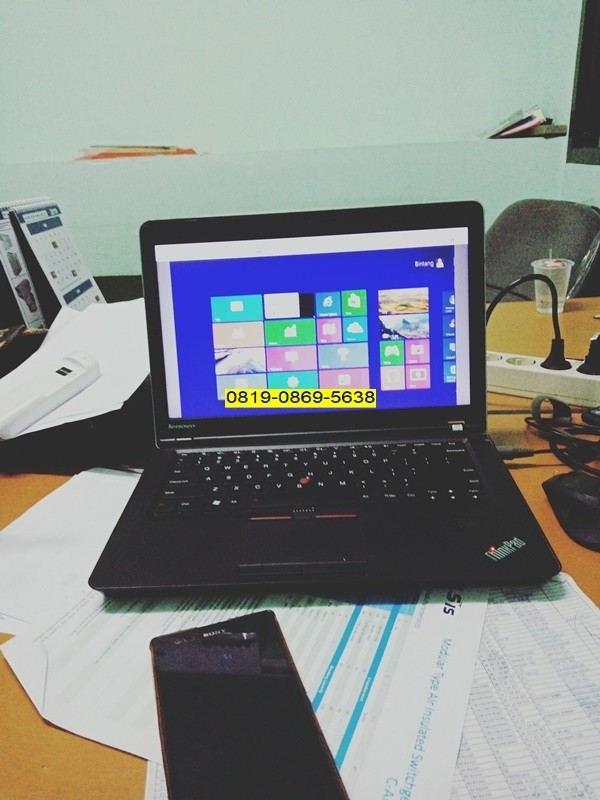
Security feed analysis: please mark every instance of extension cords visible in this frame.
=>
[485,350,600,409]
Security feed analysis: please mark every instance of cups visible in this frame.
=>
[530,258,575,315]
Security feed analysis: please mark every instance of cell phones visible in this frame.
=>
[151,609,350,800]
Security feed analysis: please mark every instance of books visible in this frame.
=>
[73,145,197,160]
[483,104,567,137]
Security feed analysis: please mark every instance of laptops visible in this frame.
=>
[88,199,564,599]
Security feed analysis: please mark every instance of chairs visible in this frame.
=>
[485,198,600,304]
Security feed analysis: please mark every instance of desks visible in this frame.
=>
[0,297,600,800]
